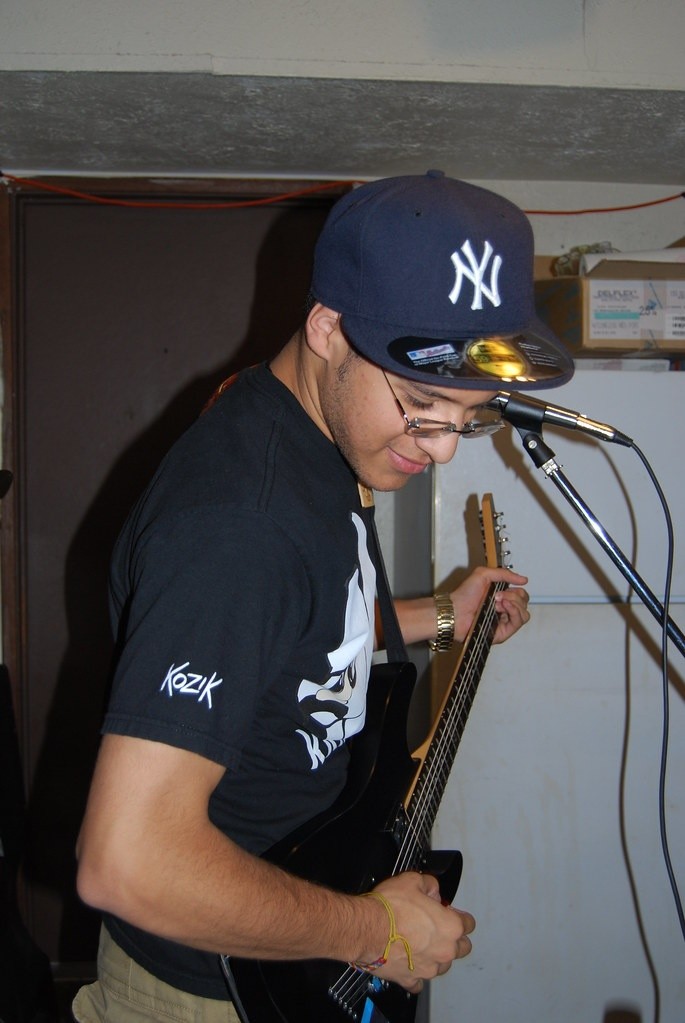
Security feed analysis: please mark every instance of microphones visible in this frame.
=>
[482,389,634,448]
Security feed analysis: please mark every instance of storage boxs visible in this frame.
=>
[526,246,685,361]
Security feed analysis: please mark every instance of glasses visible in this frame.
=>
[380,365,507,439]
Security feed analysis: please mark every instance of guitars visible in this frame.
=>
[213,490,515,1023]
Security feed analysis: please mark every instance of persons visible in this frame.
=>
[70,172,574,1023]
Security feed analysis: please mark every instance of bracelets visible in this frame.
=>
[347,891,413,973]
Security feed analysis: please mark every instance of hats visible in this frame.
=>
[309,170,574,392]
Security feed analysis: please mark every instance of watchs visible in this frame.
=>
[427,593,455,653]
